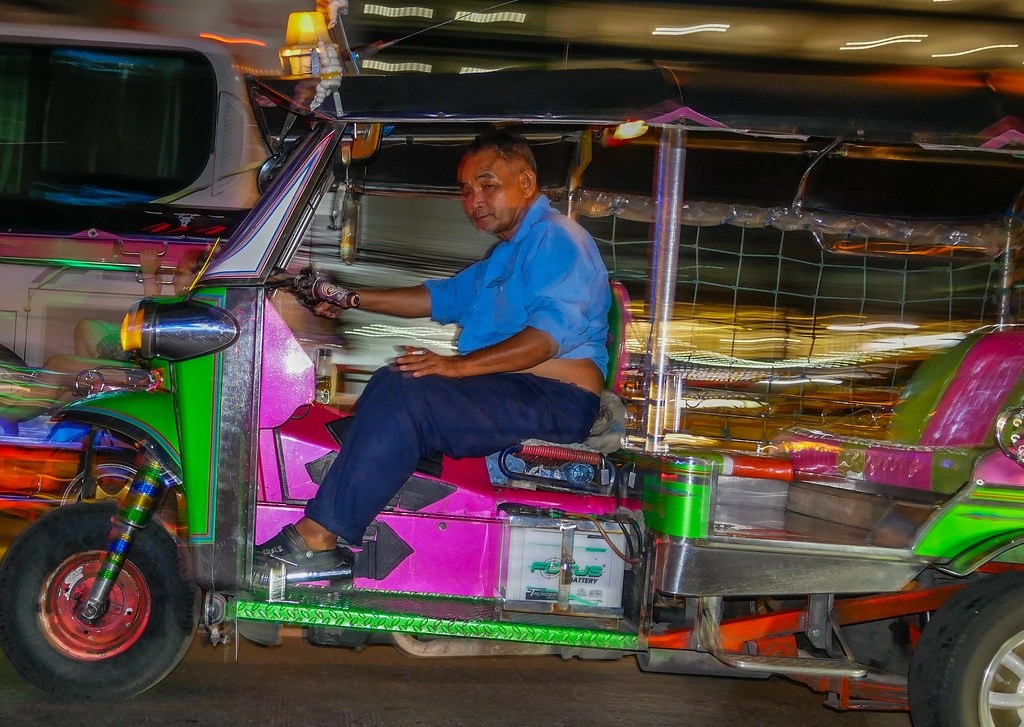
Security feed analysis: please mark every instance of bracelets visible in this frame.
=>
[142,275,157,279]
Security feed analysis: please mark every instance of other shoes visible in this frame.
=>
[252,522,353,584]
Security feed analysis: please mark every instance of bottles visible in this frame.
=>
[314,348,338,405]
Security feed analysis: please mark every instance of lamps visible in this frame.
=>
[281,12,340,58]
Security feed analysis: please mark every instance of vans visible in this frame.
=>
[0,4,300,370]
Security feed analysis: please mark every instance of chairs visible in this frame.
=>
[499,280,631,499]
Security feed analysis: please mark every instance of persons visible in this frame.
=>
[0,247,212,437]
[250,130,615,588]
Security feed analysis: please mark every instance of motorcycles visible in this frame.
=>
[2,1,1024,727]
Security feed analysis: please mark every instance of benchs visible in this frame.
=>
[769,327,1024,551]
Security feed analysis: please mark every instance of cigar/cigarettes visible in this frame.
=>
[407,350,427,355]
